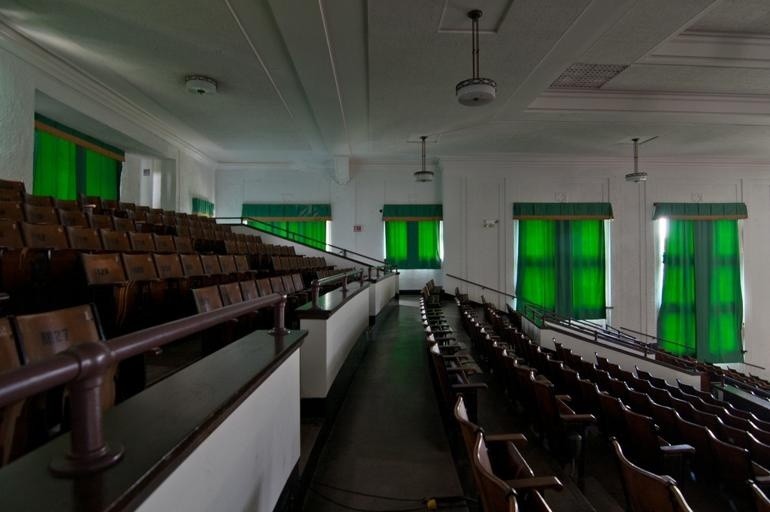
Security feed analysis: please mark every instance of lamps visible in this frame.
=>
[456,9,496,106]
[414,136,434,182]
[184,74,216,97]
[625,138,648,184]
